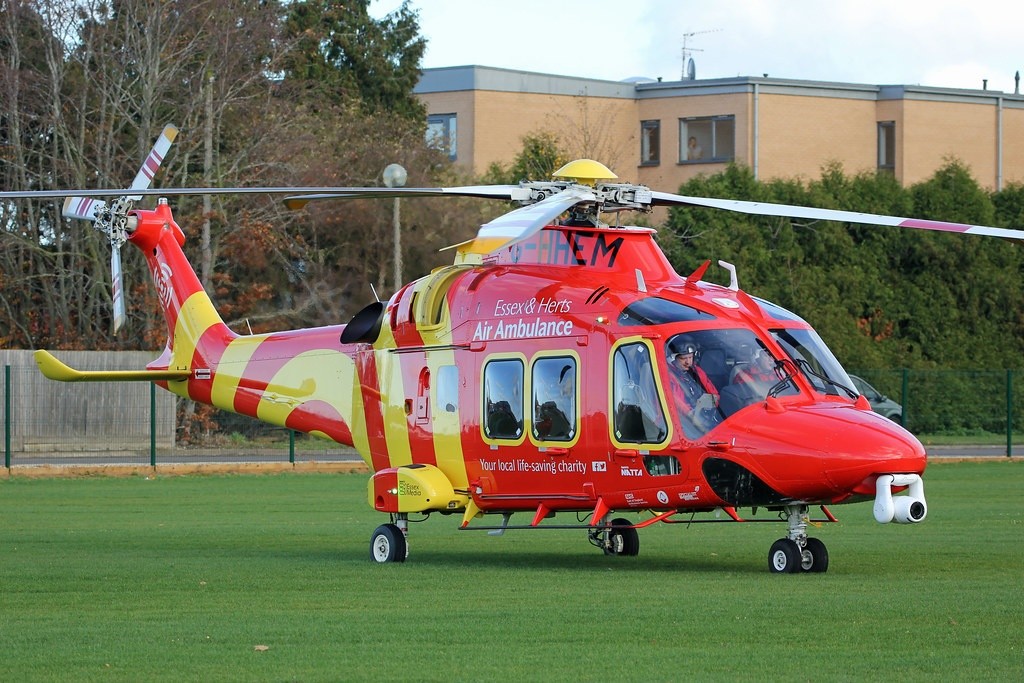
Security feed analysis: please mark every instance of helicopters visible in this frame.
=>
[0,124,1023,575]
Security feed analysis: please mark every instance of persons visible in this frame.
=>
[687,137,703,159]
[650,334,723,437]
[732,346,785,385]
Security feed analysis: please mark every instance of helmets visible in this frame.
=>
[666,334,697,356]
[750,345,768,366]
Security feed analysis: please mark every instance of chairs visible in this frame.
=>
[727,341,761,385]
[639,357,702,439]
[699,349,728,391]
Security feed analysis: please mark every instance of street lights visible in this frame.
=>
[381,164,411,292]
